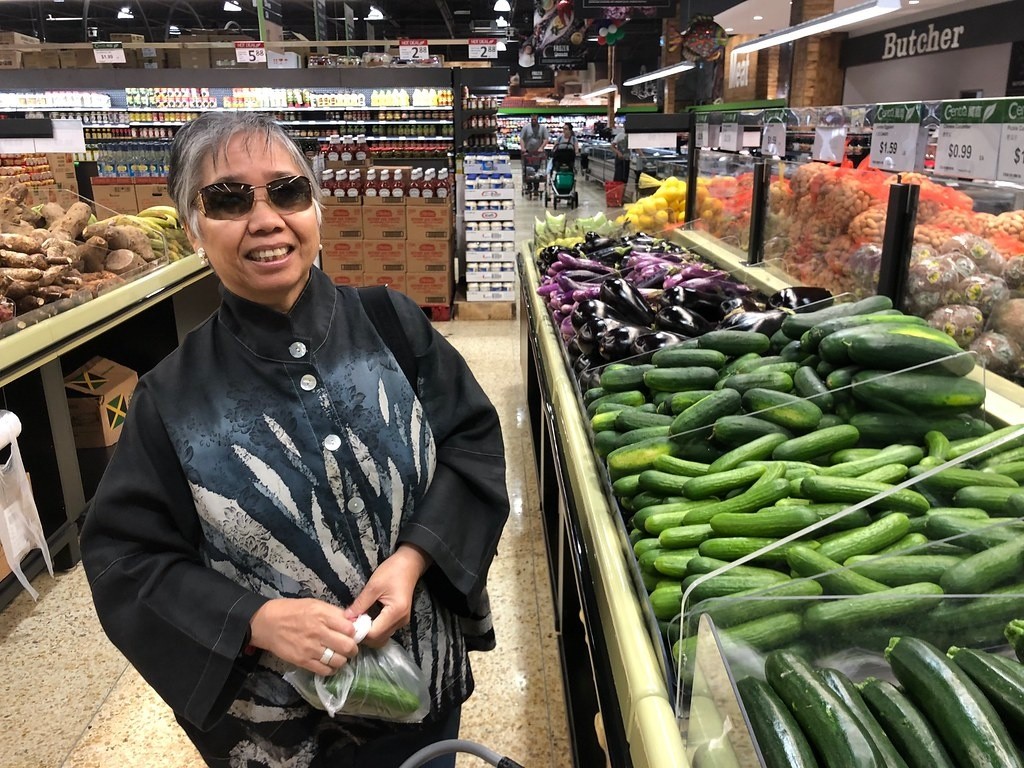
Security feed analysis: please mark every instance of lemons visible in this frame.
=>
[615,178,722,232]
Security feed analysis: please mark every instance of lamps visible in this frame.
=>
[221,1,512,27]
[577,0,903,100]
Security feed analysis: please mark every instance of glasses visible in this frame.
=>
[190,175,313,220]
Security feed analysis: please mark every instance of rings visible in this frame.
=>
[320,649,334,664]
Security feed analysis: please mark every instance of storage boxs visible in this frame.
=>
[90,158,452,307]
[0,471,34,582]
[63,357,139,448]
[0,27,310,70]
[24,152,79,208]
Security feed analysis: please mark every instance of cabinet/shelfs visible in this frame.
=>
[514,109,1024,768]
[0,188,222,613]
[498,106,657,154]
[0,67,510,168]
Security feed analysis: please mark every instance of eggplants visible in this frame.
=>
[536,230,836,383]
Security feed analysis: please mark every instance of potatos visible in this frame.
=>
[0,183,157,324]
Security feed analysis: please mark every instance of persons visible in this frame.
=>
[80,112,510,768]
[612,122,647,186]
[520,115,549,200]
[526,160,547,195]
[847,147,867,169]
[519,44,534,67]
[553,123,578,205]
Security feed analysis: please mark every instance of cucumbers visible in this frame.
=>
[307,660,420,713]
[584,295,994,461]
[733,618,1024,768]
[608,427,1024,701]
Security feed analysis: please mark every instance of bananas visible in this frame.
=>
[87,205,194,263]
[536,236,584,257]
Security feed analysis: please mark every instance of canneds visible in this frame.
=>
[125,88,218,108]
[274,111,302,121]
[129,112,198,138]
[223,87,287,108]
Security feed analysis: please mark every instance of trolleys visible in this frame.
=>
[520,149,547,200]
[544,143,579,210]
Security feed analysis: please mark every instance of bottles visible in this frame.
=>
[0,90,453,198]
[463,86,498,152]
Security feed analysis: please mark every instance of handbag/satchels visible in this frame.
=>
[282,614,430,723]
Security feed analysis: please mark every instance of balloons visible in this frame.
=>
[598,37,606,45]
[608,24,617,34]
[606,34,615,45]
[615,20,621,27]
[615,29,624,40]
[599,28,608,36]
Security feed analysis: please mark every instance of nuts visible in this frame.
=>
[781,162,1024,297]
